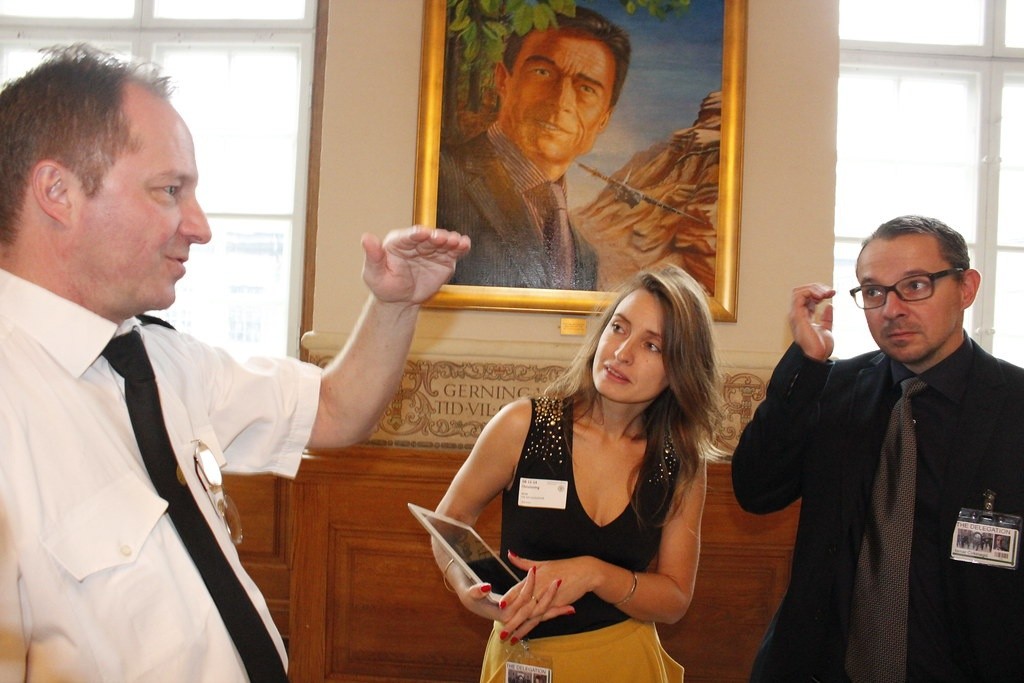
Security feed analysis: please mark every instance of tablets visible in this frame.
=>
[407,503,521,603]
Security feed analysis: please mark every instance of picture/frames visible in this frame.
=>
[410,0,749,323]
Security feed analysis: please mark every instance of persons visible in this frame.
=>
[730,214,1024,683]
[432,249,734,683]
[2,40,473,683]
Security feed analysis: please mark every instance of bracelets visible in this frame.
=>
[612,569,638,607]
[443,557,456,593]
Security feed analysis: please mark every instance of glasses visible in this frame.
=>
[850,267,964,310]
[190,439,244,545]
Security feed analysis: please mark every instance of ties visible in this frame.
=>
[102,331,289,683]
[843,377,929,683]
